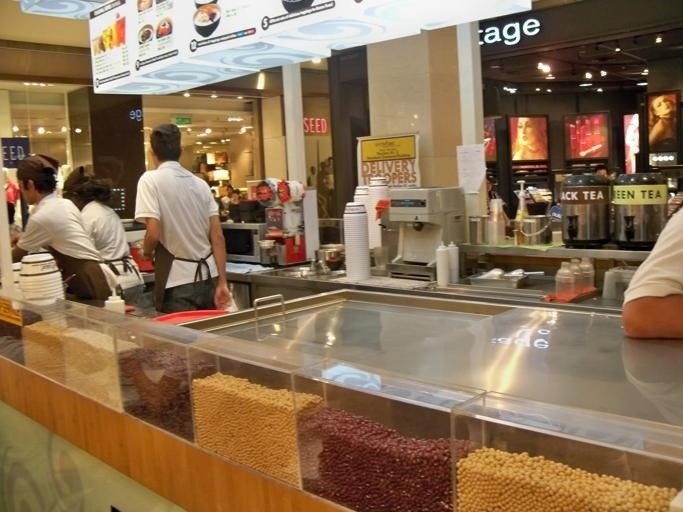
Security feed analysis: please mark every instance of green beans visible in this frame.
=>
[0,309,677,512]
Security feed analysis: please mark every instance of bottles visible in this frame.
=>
[436,240,448,288]
[488,198,506,246]
[555,255,596,299]
[448,241,460,284]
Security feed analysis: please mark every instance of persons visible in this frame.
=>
[306,157,336,218]
[512,117,548,160]
[649,94,678,150]
[622,199,683,340]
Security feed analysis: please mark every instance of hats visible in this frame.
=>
[18,153,58,180]
[63,166,96,198]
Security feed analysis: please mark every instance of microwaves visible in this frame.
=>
[220,222,269,264]
